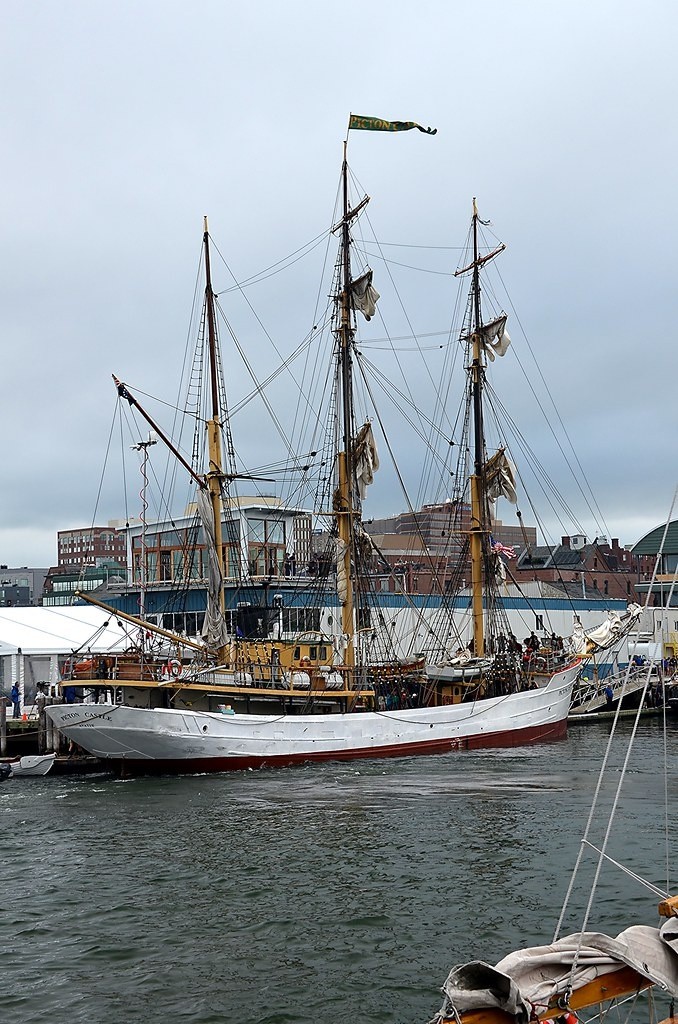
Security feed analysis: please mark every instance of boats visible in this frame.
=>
[426,657,493,683]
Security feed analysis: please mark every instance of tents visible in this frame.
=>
[0,606,204,717]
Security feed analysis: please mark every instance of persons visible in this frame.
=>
[632,655,678,677]
[605,684,613,712]
[284,552,324,577]
[11,680,22,719]
[468,631,563,652]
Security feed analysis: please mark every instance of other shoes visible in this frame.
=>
[13,716,20,719]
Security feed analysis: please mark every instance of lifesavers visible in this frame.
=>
[534,657,547,672]
[169,660,182,677]
[299,656,312,671]
[60,657,77,676]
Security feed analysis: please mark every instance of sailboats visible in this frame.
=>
[42,109,678,774]
